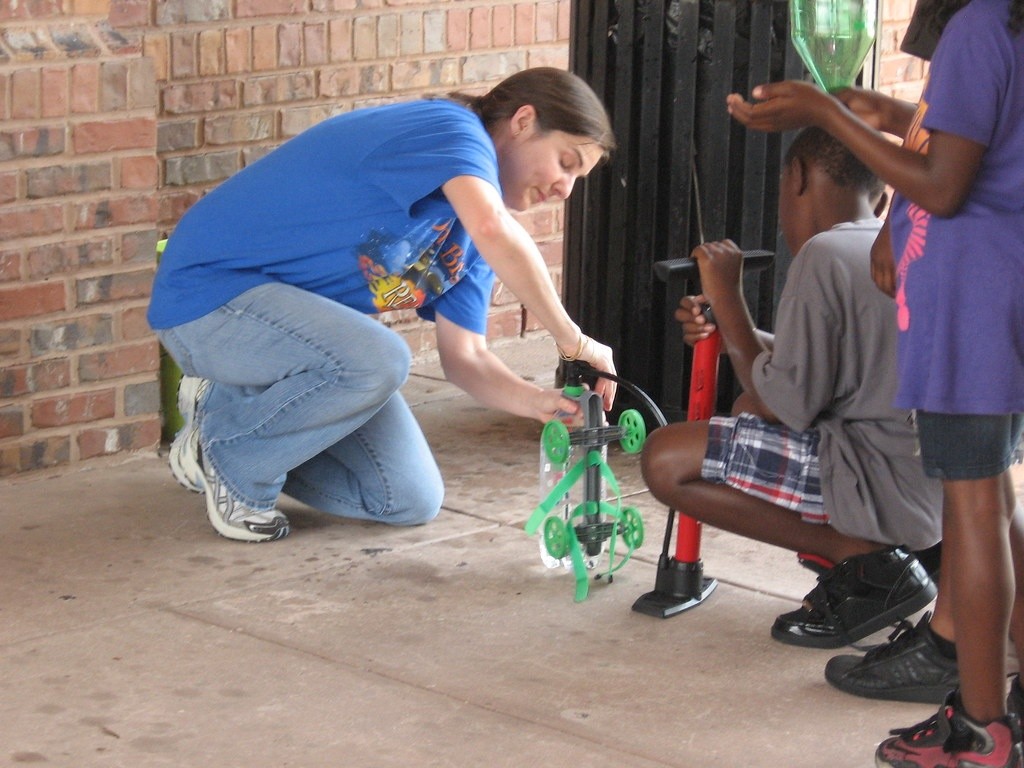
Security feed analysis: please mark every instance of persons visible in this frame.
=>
[146,66,618,544]
[639,0,1024,768]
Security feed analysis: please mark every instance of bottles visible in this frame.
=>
[787,0,876,97]
[538,387,610,572]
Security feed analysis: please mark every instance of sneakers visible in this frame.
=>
[169,374,209,494]
[1006,672,1024,768]
[771,547,938,649]
[180,427,291,542]
[824,611,961,704]
[874,687,1024,768]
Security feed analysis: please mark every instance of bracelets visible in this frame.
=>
[556,334,584,361]
